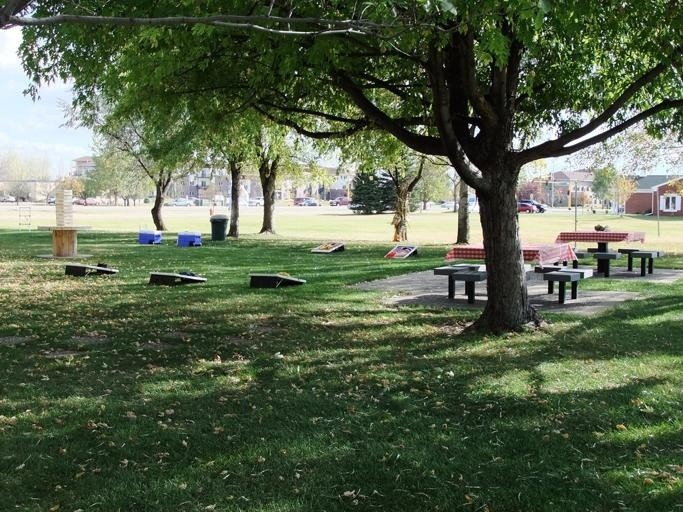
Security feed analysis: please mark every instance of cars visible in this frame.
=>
[300,200,319,207]
[516,203,538,212]
[0,190,268,209]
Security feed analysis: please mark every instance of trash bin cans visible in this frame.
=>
[210,214,229,241]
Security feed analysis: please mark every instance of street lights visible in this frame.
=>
[564,158,572,209]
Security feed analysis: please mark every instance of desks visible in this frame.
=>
[445,243,578,270]
[37,226,94,260]
[555,230,645,277]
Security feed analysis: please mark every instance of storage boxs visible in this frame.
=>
[139,229,161,244]
[177,230,202,247]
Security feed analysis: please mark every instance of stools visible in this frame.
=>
[570,247,665,276]
[434,264,593,304]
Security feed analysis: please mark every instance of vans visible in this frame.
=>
[294,198,311,205]
[441,201,455,208]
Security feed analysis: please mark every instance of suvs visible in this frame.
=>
[517,200,546,213]
[329,196,347,206]
